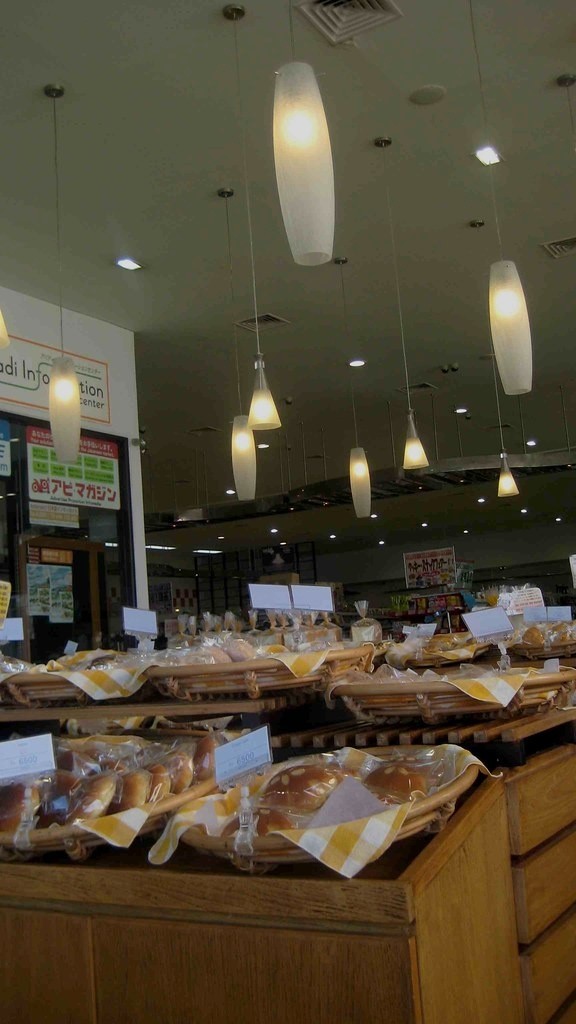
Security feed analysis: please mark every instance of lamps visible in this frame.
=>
[375,139,431,472]
[221,4,282,431]
[467,0,531,397]
[469,218,521,497]
[331,256,373,518]
[42,83,81,462]
[218,187,261,502]
[272,1,337,266]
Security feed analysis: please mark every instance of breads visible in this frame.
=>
[221,761,431,837]
[0,731,252,834]
[392,620,575,650]
[1,621,383,672]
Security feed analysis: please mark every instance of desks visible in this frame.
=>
[0,697,288,727]
[272,710,576,770]
